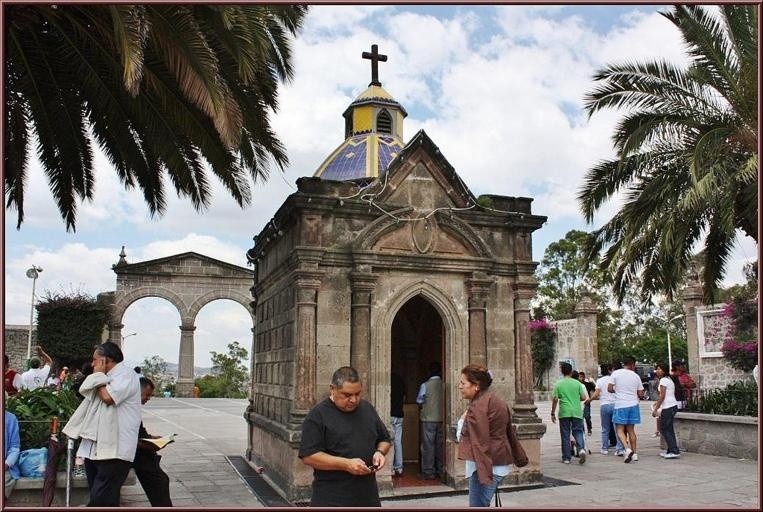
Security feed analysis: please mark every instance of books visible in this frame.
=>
[140,433,179,449]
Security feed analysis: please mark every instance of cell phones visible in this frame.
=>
[367,464,378,471]
[101,359,109,365]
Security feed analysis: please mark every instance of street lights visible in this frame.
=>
[24,263,42,357]
[649,311,685,374]
[123,331,137,339]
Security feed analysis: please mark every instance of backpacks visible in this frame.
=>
[670,375,685,401]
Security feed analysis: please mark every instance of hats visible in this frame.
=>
[672,360,687,367]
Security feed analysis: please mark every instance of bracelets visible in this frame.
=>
[376,450,385,456]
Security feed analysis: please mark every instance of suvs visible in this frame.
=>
[597,363,656,401]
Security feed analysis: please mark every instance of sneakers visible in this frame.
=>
[578,448,585,464]
[664,451,679,458]
[631,454,637,461]
[623,448,633,462]
[600,449,608,454]
[561,460,570,463]
[614,449,623,456]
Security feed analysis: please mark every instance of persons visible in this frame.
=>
[652,364,681,458]
[134,367,145,377]
[135,378,172,507]
[298,366,392,508]
[76,342,141,507]
[456,365,529,508]
[391,372,407,475]
[670,361,695,408]
[550,356,643,463]
[5,411,21,501]
[4,346,85,396]
[416,361,442,479]
